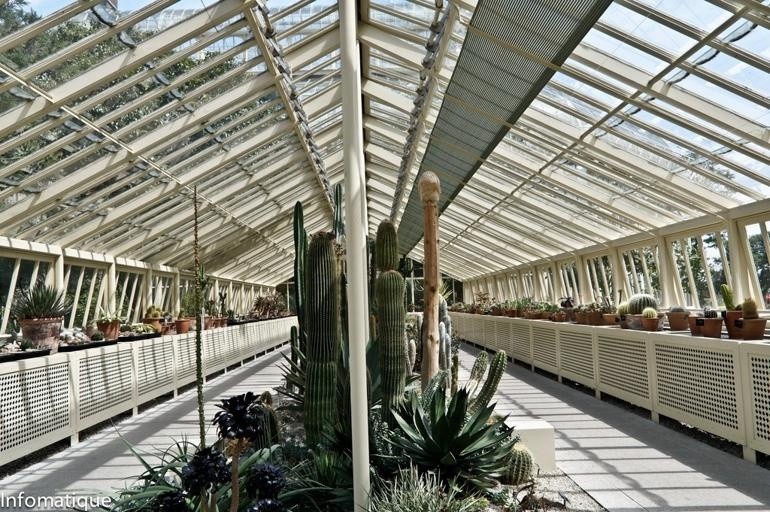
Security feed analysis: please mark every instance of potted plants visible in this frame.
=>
[1,279,294,360]
